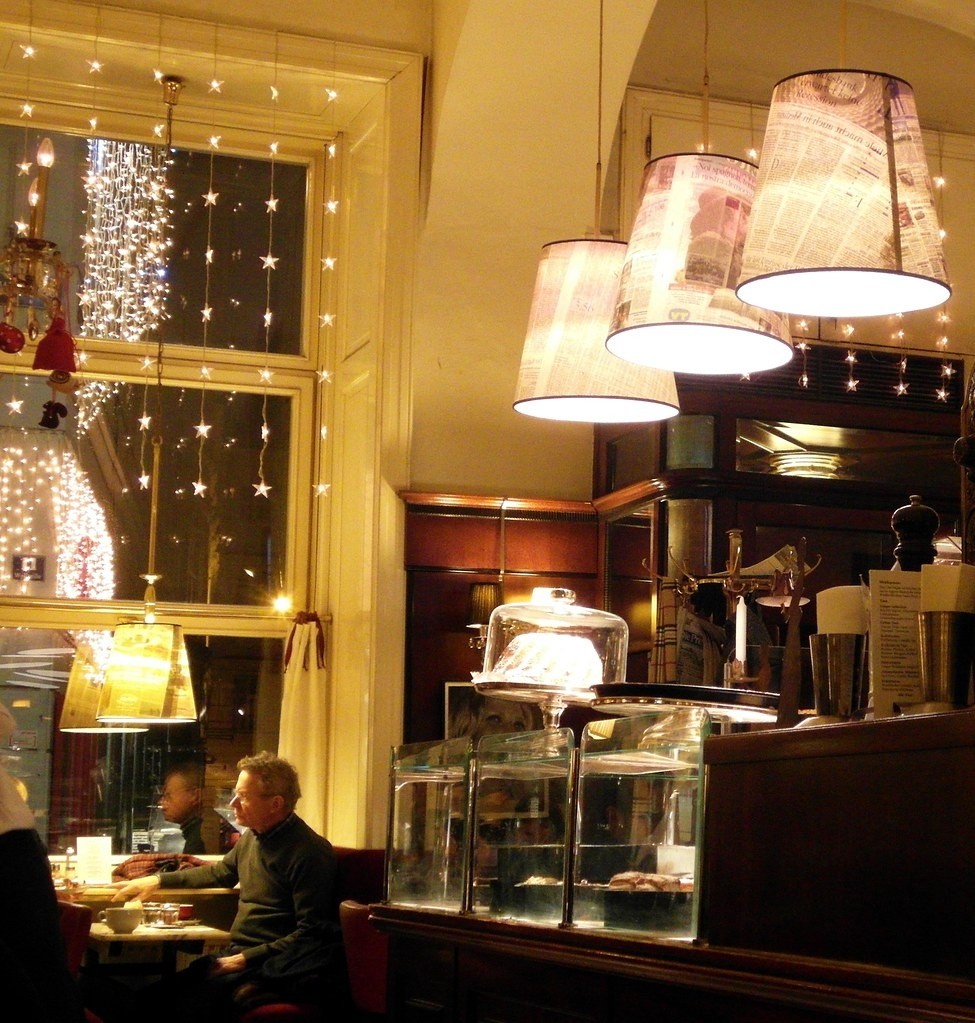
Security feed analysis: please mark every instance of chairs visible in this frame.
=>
[243,848,388,1023]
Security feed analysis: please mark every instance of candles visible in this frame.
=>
[736,594,746,661]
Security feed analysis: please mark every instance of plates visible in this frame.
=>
[174,919,201,925]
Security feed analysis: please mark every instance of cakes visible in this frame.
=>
[491,633,604,689]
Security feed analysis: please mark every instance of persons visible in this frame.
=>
[490,793,566,908]
[104,749,348,1023]
[0,764,88,1023]
[158,763,240,855]
[449,688,544,813]
[582,769,674,882]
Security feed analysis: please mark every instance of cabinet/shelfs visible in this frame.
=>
[384,709,714,939]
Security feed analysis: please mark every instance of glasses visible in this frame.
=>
[232,789,275,802]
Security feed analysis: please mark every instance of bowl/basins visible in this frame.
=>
[179,904,194,920]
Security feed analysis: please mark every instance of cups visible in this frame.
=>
[917,611,975,710]
[808,633,869,718]
[142,903,162,925]
[161,903,181,925]
[97,908,143,934]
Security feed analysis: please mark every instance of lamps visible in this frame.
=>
[512,0,679,425]
[60,632,150,734]
[735,0,952,317]
[605,0,795,379]
[95,70,201,724]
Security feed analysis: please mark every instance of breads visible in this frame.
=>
[607,870,695,892]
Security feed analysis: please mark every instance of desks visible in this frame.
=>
[73,886,241,904]
[91,921,231,955]
